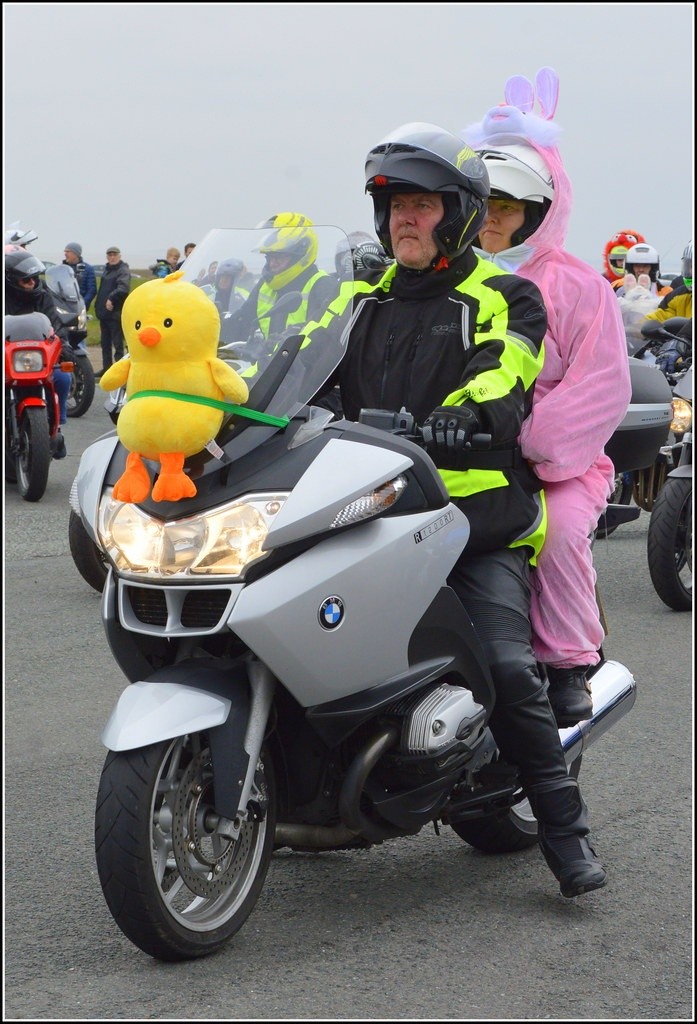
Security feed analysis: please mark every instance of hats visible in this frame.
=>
[65,242,82,257]
[106,247,120,254]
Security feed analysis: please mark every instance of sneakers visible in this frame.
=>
[538,831,609,898]
[548,663,594,722]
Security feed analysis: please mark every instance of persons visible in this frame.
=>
[220,212,329,347]
[5,229,76,423]
[192,258,252,313]
[244,66,632,898]
[152,243,196,277]
[61,243,97,347]
[603,230,692,372]
[94,246,130,378]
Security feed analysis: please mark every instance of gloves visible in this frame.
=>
[59,347,77,366]
[654,340,687,375]
[422,404,479,455]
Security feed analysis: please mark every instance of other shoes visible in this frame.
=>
[52,428,66,460]
[94,368,108,377]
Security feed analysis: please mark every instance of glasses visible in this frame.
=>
[17,275,38,284]
[266,252,291,265]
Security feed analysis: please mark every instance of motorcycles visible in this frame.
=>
[44,265,95,419]
[103,291,302,425]
[5,309,78,503]
[597,281,691,612]
[66,224,674,962]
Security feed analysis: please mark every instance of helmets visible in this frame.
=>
[607,245,627,277]
[470,141,554,207]
[625,243,661,283]
[362,122,491,258]
[5,245,47,304]
[214,257,247,290]
[5,229,38,248]
[252,212,318,291]
[334,230,378,277]
[680,240,693,291]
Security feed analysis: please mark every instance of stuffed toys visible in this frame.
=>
[98,271,250,504]
